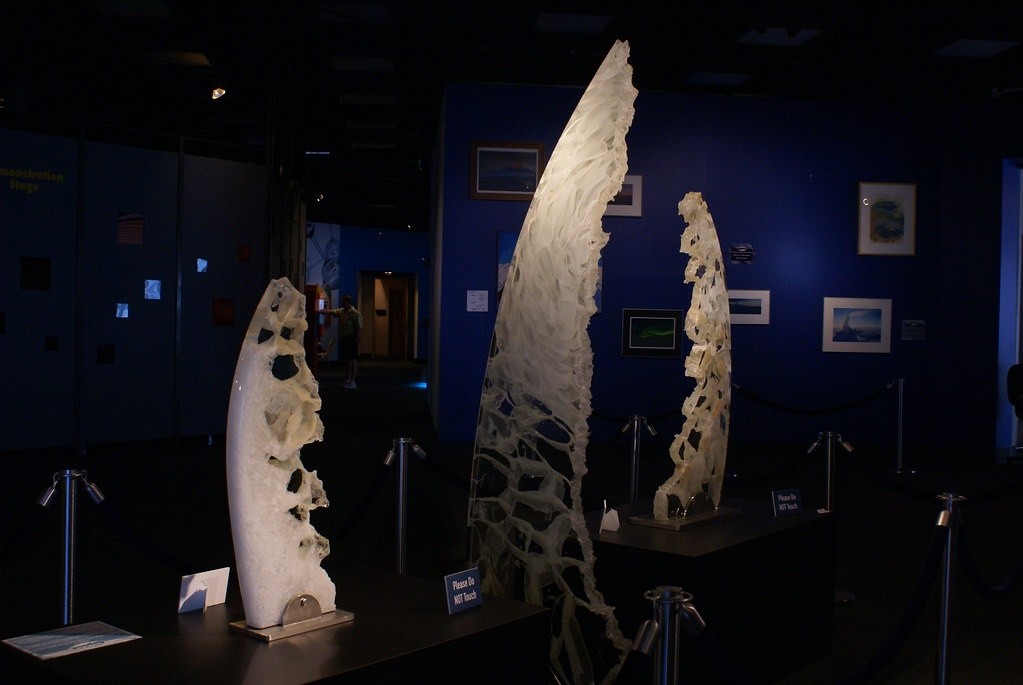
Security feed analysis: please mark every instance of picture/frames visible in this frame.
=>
[468,140,546,202]
[603,175,643,218]
[822,297,892,354]
[494,227,604,316]
[728,290,772,326]
[857,181,917,256]
[621,308,684,360]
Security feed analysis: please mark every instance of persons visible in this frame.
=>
[312,337,333,357]
[315,295,364,389]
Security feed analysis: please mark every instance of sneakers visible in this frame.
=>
[338,379,357,389]
